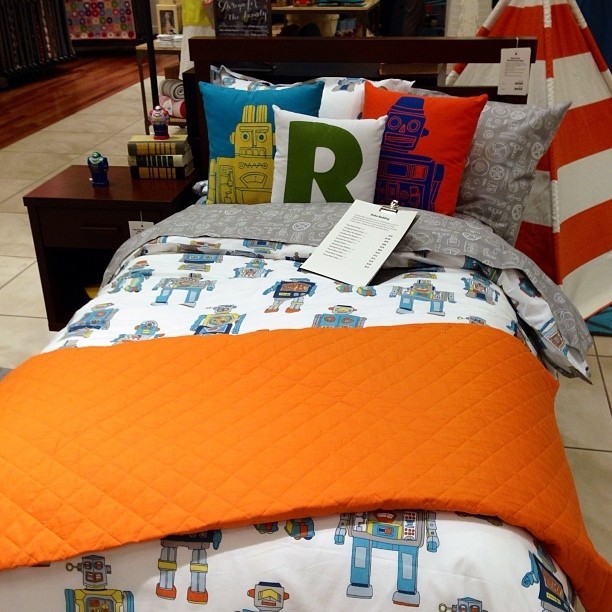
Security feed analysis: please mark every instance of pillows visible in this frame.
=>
[360,82,489,214]
[195,80,323,204]
[456,100,572,248]
[270,104,387,204]
[207,62,415,119]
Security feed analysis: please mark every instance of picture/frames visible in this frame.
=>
[155,4,181,34]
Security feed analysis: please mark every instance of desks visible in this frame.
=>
[134,40,182,136]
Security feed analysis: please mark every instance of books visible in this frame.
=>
[128,134,191,156]
[128,149,194,167]
[130,161,195,180]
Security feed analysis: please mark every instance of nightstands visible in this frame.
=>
[22,164,197,332]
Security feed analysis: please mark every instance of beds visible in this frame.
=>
[0,35,611,612]
